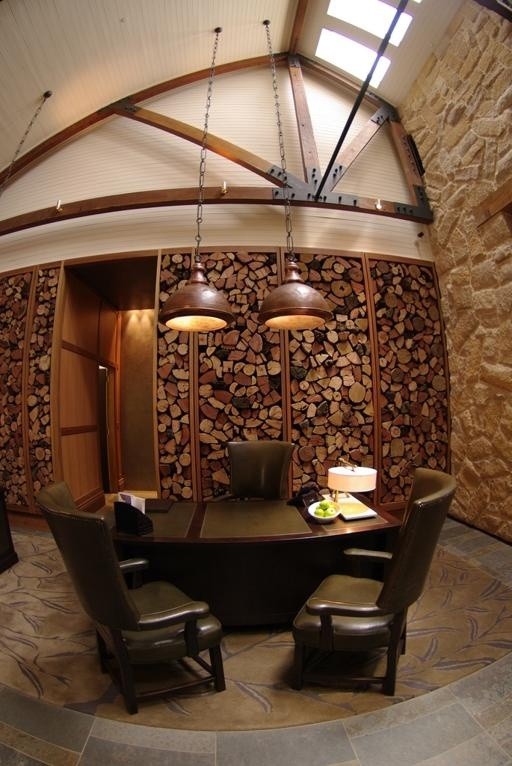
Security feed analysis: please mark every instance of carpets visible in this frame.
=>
[0,530,512,731]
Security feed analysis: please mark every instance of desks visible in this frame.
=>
[89,501,400,624]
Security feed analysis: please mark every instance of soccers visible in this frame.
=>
[314,500,336,517]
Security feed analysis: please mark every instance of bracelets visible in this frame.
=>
[307,500,342,523]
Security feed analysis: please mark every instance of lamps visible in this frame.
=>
[256,20,329,332]
[158,28,237,332]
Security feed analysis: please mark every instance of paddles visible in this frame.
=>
[0,530,512,731]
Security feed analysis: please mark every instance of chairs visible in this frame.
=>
[294,467,459,697]
[38,483,226,713]
[229,440,295,496]
